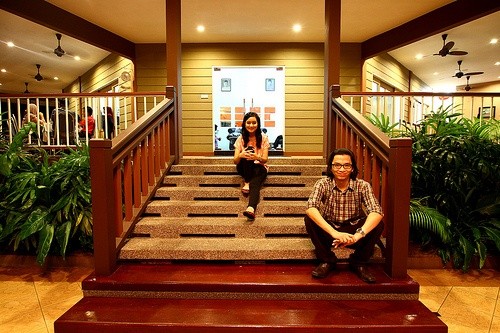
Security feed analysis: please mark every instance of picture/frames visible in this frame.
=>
[479,106,496,119]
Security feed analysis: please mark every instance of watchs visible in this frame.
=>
[355,227,366,237]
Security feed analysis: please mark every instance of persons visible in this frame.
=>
[50,98,81,145]
[77,106,95,138]
[22,103,46,146]
[267,79,274,90]
[101,106,114,140]
[233,111,270,220]
[222,79,231,91]
[303,147,384,283]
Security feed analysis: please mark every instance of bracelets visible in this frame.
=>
[257,156,263,162]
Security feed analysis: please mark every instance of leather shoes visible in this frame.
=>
[351,265,376,282]
[311,262,337,277]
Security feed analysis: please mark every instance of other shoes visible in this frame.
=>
[243,211,255,220]
[242,185,250,193]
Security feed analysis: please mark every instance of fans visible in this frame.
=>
[433,34,469,57]
[458,76,472,91]
[42,33,73,60]
[28,64,52,82]
[451,61,484,79]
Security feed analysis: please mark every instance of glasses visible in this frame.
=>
[331,163,353,169]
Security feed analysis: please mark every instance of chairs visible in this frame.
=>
[7,112,75,145]
